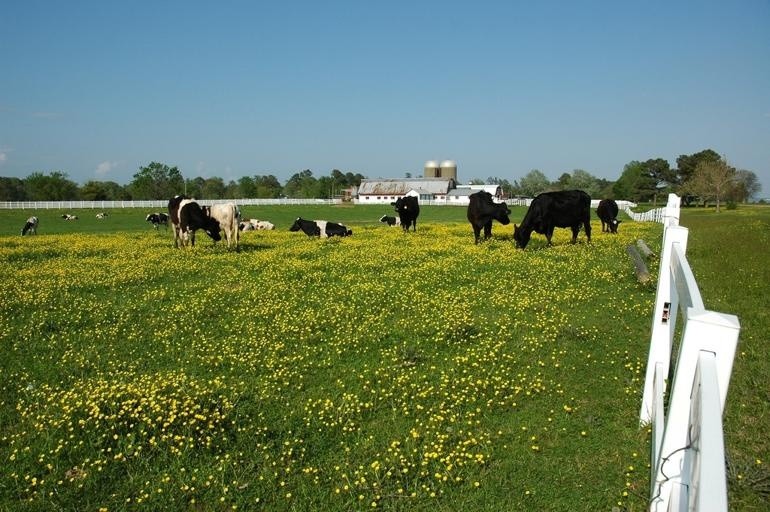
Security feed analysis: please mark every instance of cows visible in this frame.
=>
[595,199,622,234]
[379,215,401,227]
[62,214,78,221]
[146,194,275,253]
[96,212,108,218]
[513,189,592,251]
[467,190,512,245]
[20,216,39,236]
[290,216,352,239]
[391,196,419,232]
